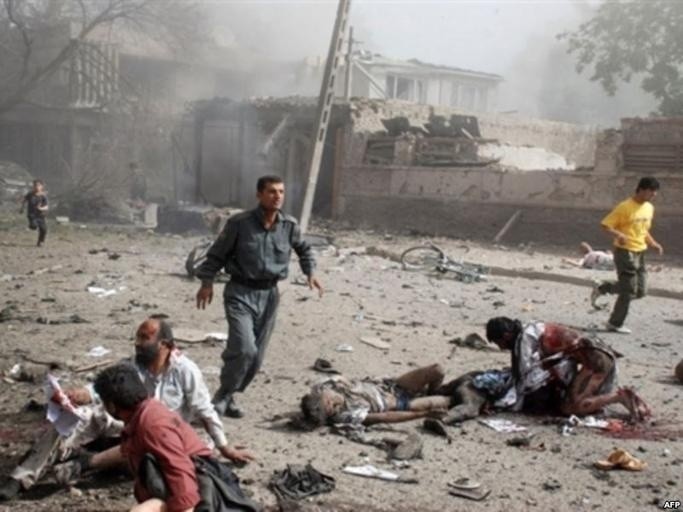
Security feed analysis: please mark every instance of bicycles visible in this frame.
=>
[400,243,493,282]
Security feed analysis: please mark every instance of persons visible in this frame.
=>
[589,176,663,335]
[122,159,147,213]
[485,316,652,425]
[51,312,257,478]
[562,240,661,272]
[196,177,324,421]
[94,361,241,510]
[18,178,49,248]
[298,364,453,427]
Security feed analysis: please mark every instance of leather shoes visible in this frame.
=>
[606,324,630,333]
[211,391,232,415]
[226,400,245,417]
[590,280,602,307]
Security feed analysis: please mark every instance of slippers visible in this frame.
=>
[593,450,648,471]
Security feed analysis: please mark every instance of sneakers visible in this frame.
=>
[424,418,449,438]
